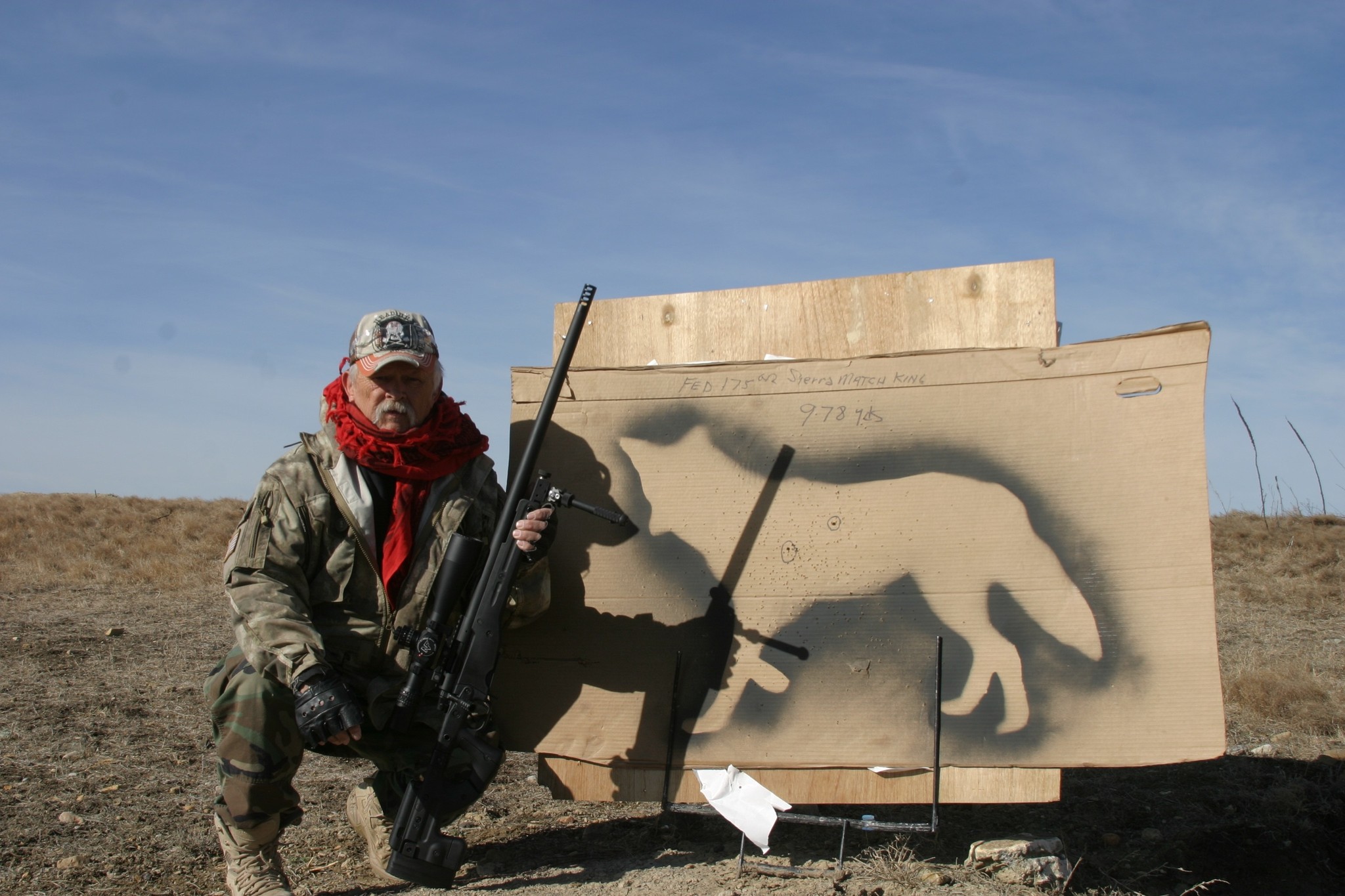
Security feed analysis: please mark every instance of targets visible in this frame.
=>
[508,255,1228,879]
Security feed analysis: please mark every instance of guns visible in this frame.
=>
[381,282,627,890]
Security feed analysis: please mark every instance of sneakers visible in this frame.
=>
[213,810,297,896]
[345,769,407,882]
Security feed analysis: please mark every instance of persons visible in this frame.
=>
[203,309,564,896]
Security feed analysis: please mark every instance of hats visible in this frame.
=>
[348,308,439,378]
